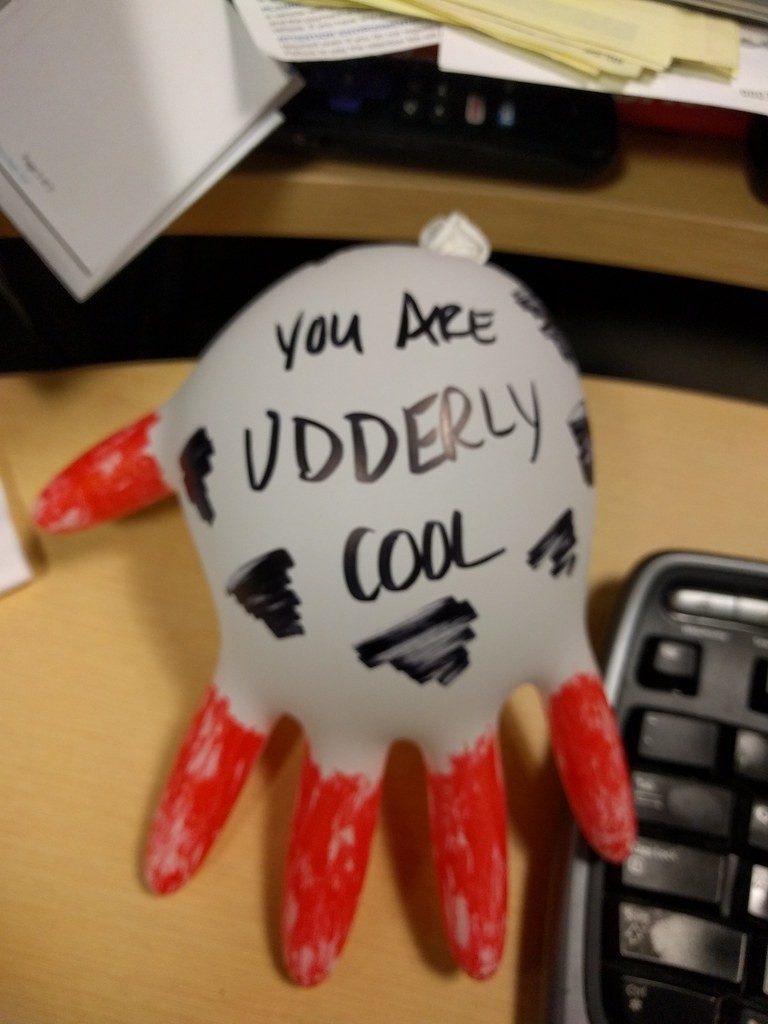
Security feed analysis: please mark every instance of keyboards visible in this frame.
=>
[538,547,768,1024]
[281,58,621,188]
